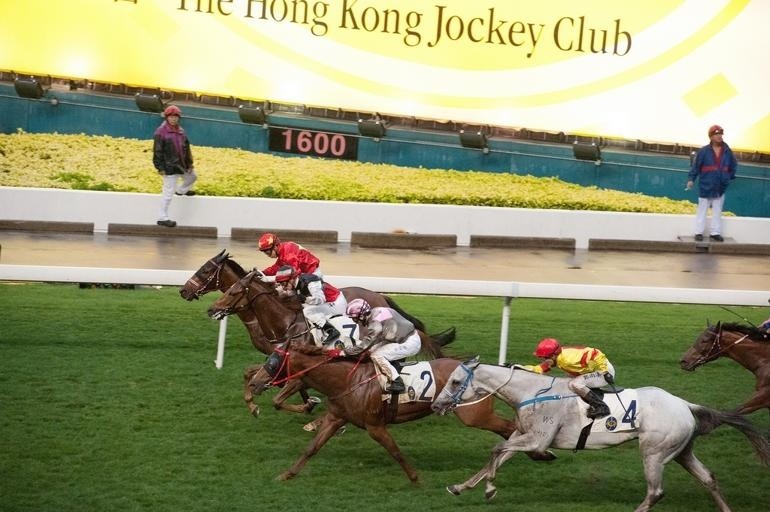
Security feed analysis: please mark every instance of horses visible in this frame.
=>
[180,249,457,404]
[679,318,770,436]
[247,337,558,486]
[431,355,770,512]
[207,269,476,419]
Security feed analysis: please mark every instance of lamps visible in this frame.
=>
[10,73,609,167]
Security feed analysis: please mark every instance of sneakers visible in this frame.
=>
[696,233,725,242]
[158,219,177,228]
[178,189,194,196]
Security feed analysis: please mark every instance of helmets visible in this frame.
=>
[347,298,371,319]
[535,337,562,358]
[275,264,299,281]
[165,103,183,121]
[708,125,723,137]
[257,233,280,251]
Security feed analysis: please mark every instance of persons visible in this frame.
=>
[687,125,738,241]
[757,299,770,332]
[257,233,323,296]
[514,337,616,418]
[326,299,422,392]
[275,265,348,345]
[153,105,197,227]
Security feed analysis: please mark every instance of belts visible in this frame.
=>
[396,329,415,343]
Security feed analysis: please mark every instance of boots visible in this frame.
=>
[584,390,611,417]
[322,323,341,344]
[386,378,406,390]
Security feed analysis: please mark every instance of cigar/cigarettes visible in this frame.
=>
[684,188,689,191]
